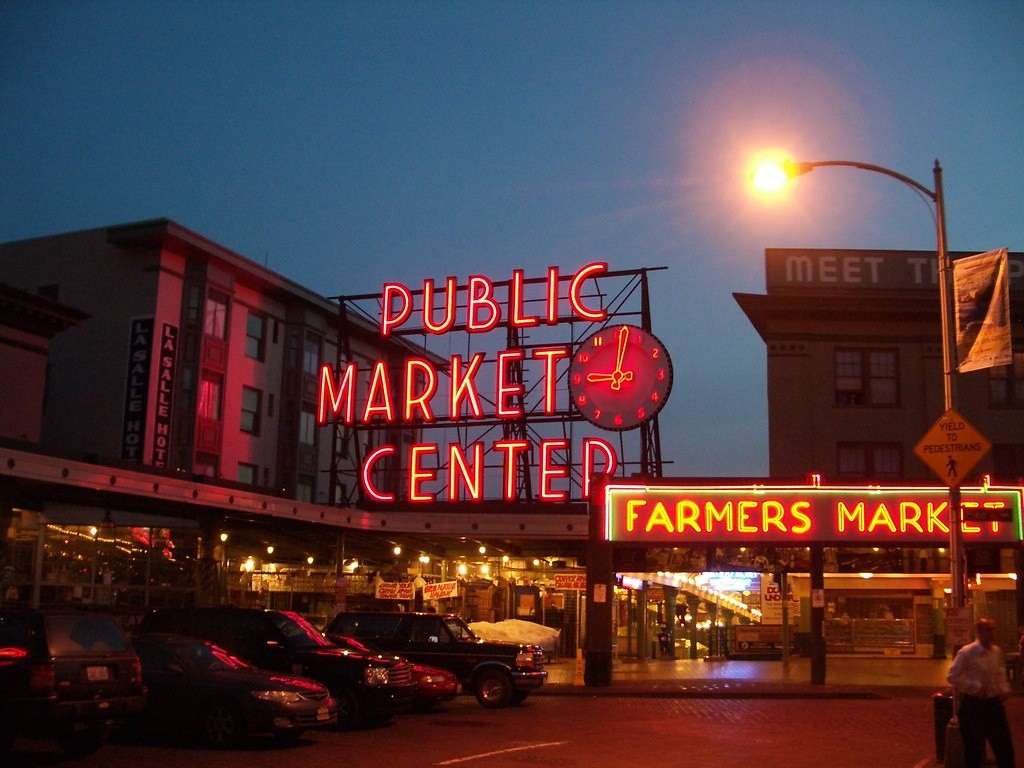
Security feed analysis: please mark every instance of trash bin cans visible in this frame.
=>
[934,690,955,759]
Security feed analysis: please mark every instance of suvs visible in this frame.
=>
[138,605,412,725]
[318,608,549,709]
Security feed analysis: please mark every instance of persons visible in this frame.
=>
[1018,626,1024,688]
[423,607,441,642]
[945,618,1015,768]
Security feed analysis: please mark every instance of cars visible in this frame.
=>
[123,635,339,748]
[0,609,148,758]
[327,634,463,709]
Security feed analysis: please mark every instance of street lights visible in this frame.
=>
[751,152,968,612]
